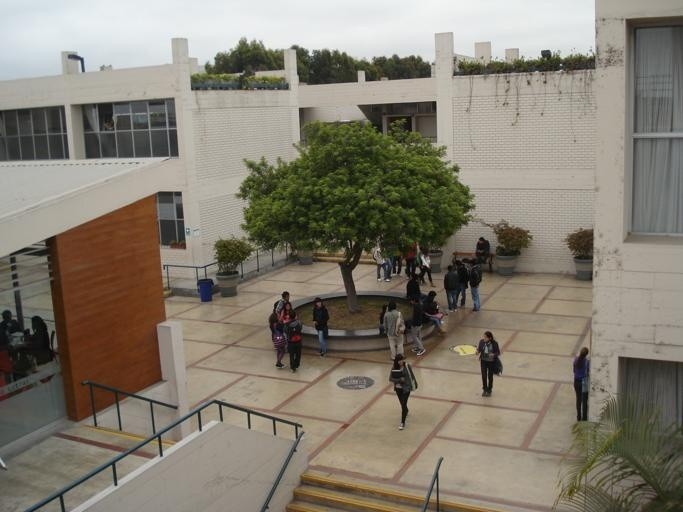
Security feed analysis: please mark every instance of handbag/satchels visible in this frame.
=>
[405,362,416,392]
[492,341,503,375]
[395,311,406,337]
[581,360,590,394]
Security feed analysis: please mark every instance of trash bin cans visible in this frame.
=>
[196,278,212,302]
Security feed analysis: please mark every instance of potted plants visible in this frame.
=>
[215,240,253,298]
[294,238,318,265]
[564,226,594,281]
[488,220,534,277]
[427,238,447,273]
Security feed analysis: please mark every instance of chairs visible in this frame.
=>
[31,330,56,366]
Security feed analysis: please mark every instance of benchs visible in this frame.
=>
[453,251,495,273]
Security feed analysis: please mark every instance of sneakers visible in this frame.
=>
[411,348,419,353]
[416,348,427,356]
[398,422,405,431]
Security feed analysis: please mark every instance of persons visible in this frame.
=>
[383,301,406,360]
[474,332,500,397]
[423,291,447,337]
[441,258,482,313]
[373,247,390,282]
[385,258,392,280]
[476,237,490,264]
[0,310,54,384]
[404,243,436,297]
[388,354,418,431]
[312,297,329,357]
[269,291,302,372]
[409,295,427,356]
[392,252,402,277]
[572,347,590,420]
[379,305,390,335]
